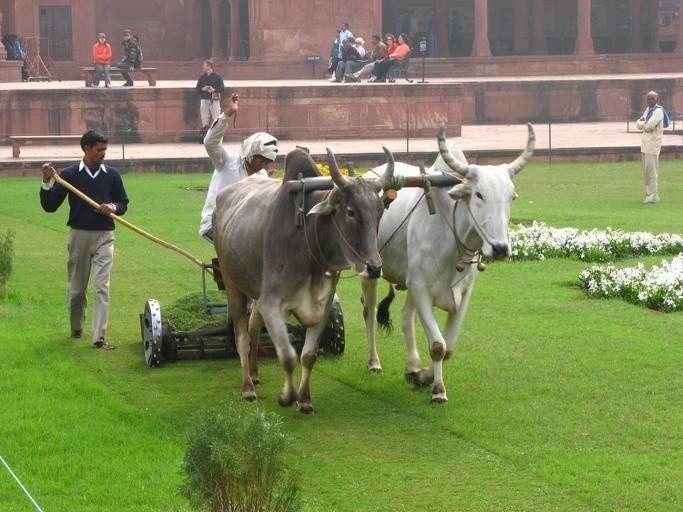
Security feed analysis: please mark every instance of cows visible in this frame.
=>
[210,146,395,416]
[355,120,537,405]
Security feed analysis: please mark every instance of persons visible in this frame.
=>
[633,89,664,204]
[400,7,465,55]
[197,90,279,243]
[89,31,113,87]
[36,128,130,351]
[323,22,411,84]
[194,58,225,129]
[117,29,144,86]
[1,32,33,82]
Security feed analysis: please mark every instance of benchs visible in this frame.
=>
[363,50,414,83]
[81,65,158,87]
[5,135,86,158]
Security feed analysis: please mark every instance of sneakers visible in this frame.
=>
[326,65,389,83]
[643,193,660,204]
[92,82,133,88]
[93,339,116,351]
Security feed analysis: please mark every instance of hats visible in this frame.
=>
[96,33,106,38]
[122,29,132,35]
